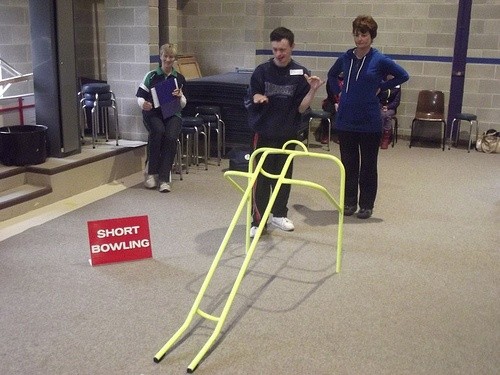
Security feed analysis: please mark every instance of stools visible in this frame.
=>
[78,83,119,149]
[450,110,478,152]
[308,111,331,151]
[172,106,225,180]
[391,117,397,147]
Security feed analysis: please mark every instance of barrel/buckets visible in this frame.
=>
[415,89,445,119]
[0,124,48,166]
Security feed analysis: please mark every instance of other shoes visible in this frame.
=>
[333,138,340,143]
[321,136,329,144]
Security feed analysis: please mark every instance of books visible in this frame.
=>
[150,76,181,118]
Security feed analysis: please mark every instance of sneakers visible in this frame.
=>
[344,205,357,216]
[250,226,267,237]
[272,216,295,230]
[159,181,173,192]
[144,175,158,189]
[357,208,372,219]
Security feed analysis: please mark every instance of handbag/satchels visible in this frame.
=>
[476,129,500,154]
[230,150,251,171]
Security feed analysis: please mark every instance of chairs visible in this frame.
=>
[411,89,445,149]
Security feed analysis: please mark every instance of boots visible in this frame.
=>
[381,128,391,149]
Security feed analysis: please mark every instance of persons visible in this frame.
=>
[314,72,401,149]
[249,27,326,238]
[136,43,187,193]
[326,15,409,219]
